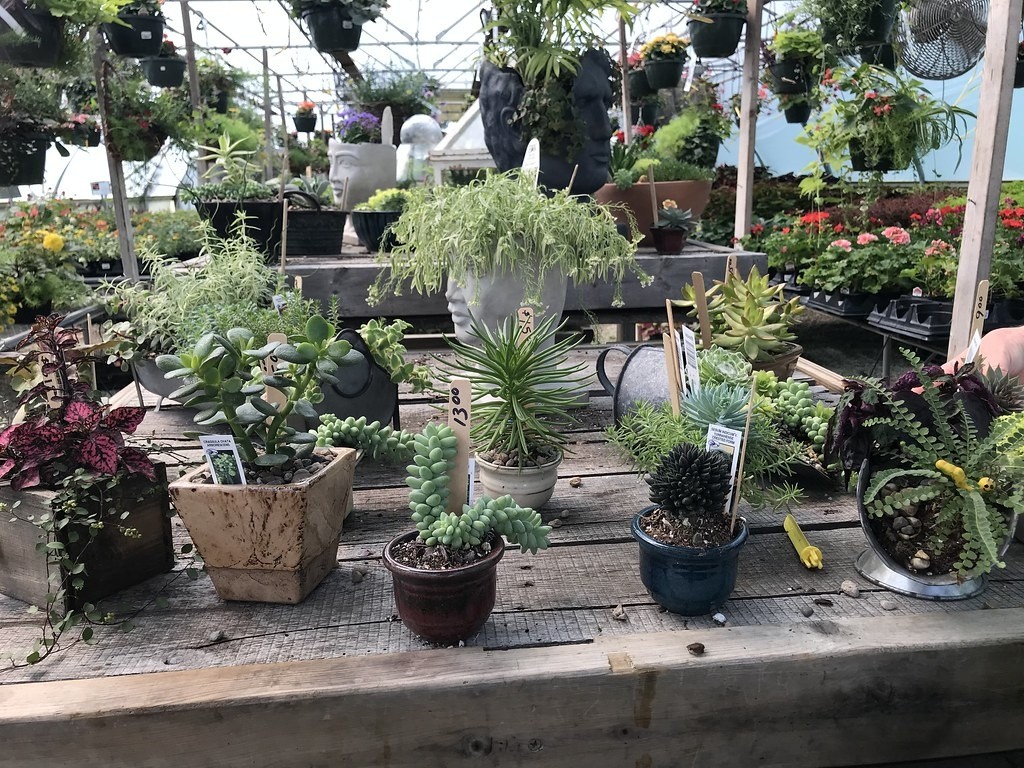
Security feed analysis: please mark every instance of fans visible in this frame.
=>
[890,0,988,81]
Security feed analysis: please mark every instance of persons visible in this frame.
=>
[328,138,396,233]
[446,237,567,373]
[480,47,613,203]
[912,326,1024,402]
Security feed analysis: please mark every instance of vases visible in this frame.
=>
[859,43,897,72]
[138,58,187,88]
[327,138,396,245]
[11,299,50,325]
[783,101,811,124]
[629,103,659,127]
[593,179,714,247]
[102,14,166,58]
[848,138,909,171]
[301,7,362,52]
[651,227,687,254]
[685,12,745,58]
[446,249,590,413]
[644,61,683,90]
[765,62,812,95]
[1,130,48,186]
[626,71,658,96]
[292,116,317,132]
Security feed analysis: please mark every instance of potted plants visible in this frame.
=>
[1,213,1024,675]
[178,129,284,265]
[278,172,348,257]
[350,186,414,254]
[477,0,642,206]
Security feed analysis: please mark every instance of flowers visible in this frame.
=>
[0,0,1024,332]
